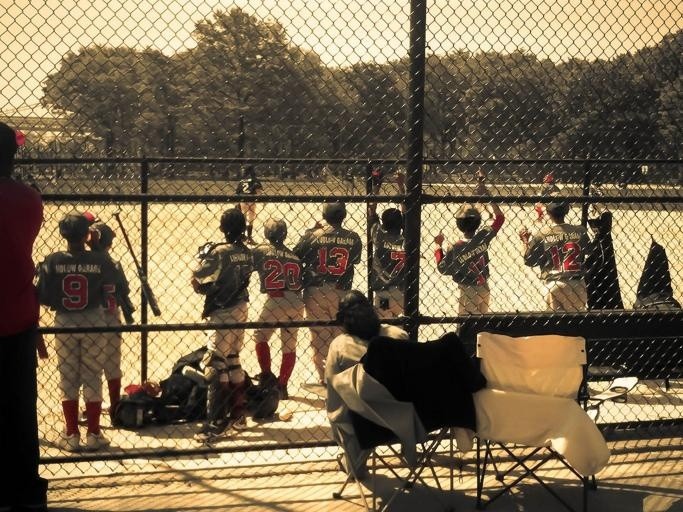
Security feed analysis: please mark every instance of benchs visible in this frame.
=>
[453,306,683,391]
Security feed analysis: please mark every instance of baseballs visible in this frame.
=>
[278,411,291,422]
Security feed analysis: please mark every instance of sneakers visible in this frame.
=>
[86,430,110,450]
[256,370,278,398]
[193,417,234,441]
[276,377,289,400]
[232,413,247,430]
[51,427,80,450]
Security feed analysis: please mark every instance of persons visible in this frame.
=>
[293,202,361,385]
[367,171,404,318]
[192,210,255,441]
[434,188,505,332]
[37,211,111,451]
[86,222,136,425]
[0,122,44,512]
[534,175,560,219]
[519,203,589,313]
[254,217,305,399]
[236,165,262,244]
[323,290,408,480]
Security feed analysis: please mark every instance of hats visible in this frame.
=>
[221,209,247,233]
[59,210,96,237]
[383,207,404,229]
[453,203,481,219]
[0,120,26,158]
[547,190,570,215]
[322,202,346,223]
[265,218,287,242]
[88,222,117,248]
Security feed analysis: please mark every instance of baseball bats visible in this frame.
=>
[112,210,161,316]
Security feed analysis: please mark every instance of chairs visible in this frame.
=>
[330,328,477,511]
[472,330,611,511]
